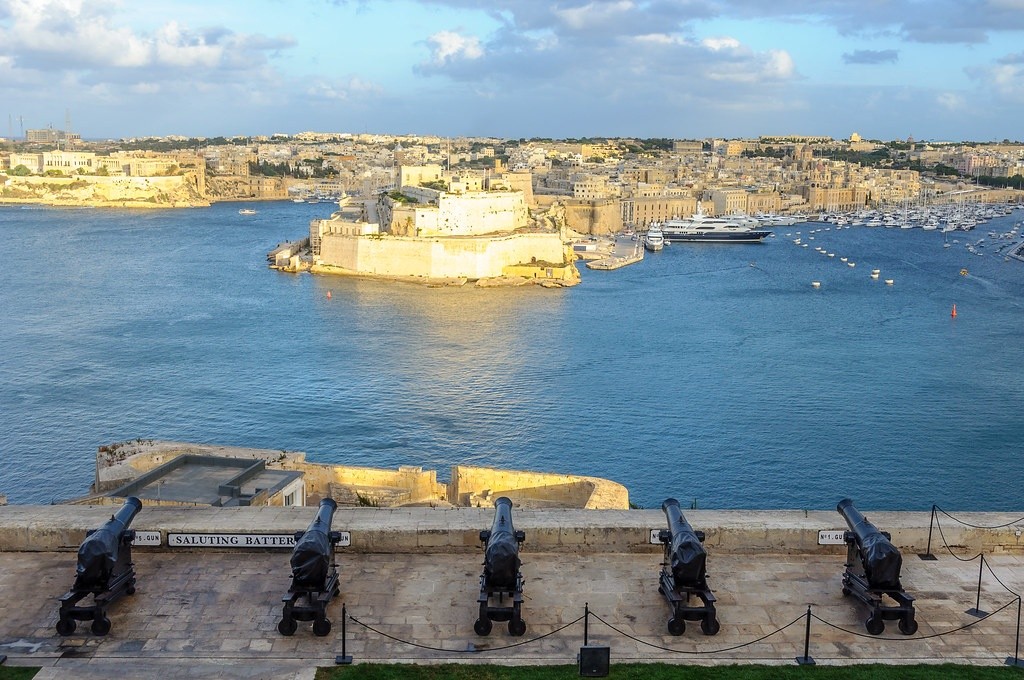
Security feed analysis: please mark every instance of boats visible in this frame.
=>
[664,240,671,246]
[663,202,897,287]
[953,230,1024,262]
[239,209,256,215]
[645,226,664,251]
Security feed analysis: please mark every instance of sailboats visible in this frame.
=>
[943,233,951,248]
[290,174,396,204]
[892,185,1024,232]
